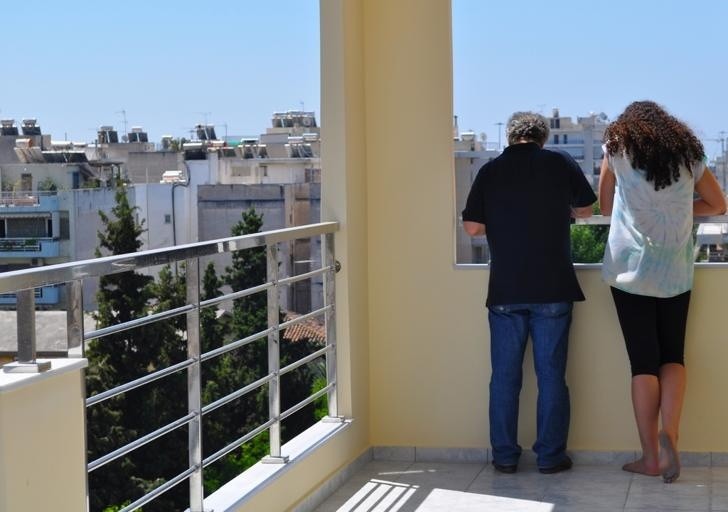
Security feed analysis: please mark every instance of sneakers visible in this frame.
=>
[539,456,572,474]
[492,458,517,474]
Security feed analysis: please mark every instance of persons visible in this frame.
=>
[460,111,597,475]
[596,100,726,484]
